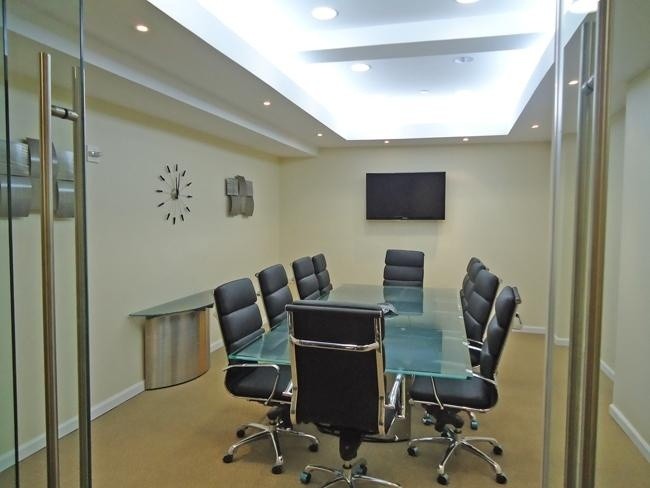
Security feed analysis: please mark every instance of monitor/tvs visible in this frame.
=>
[365,172,445,220]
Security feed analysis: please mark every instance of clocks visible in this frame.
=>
[153,162,193,225]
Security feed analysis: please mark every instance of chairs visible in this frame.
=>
[421,256,503,433]
[280,298,406,487]
[292,256,321,299]
[312,252,333,294]
[212,277,322,476]
[407,286,521,486]
[383,248,424,287]
[255,263,293,330]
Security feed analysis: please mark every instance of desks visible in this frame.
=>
[128,290,215,392]
[234,285,474,445]
